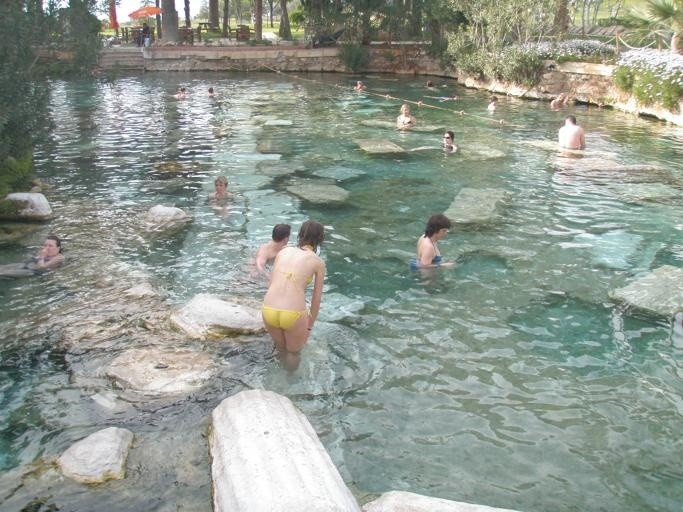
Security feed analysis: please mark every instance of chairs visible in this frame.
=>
[306,29,346,48]
[115,27,156,47]
[226,24,256,42]
[178,26,201,46]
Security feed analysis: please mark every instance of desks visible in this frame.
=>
[199,23,212,33]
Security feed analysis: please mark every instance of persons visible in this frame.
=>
[425,79,445,94]
[414,213,454,278]
[205,86,219,104]
[261,219,326,372]
[422,92,460,103]
[550,92,570,113]
[396,102,417,133]
[441,129,458,157]
[25,234,66,270]
[136,22,151,50]
[485,96,500,120]
[173,87,192,105]
[354,80,369,94]
[206,176,238,205]
[255,224,293,277]
[559,115,587,152]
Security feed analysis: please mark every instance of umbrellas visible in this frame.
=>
[127,3,167,31]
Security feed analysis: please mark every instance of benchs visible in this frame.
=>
[199,27,220,33]
